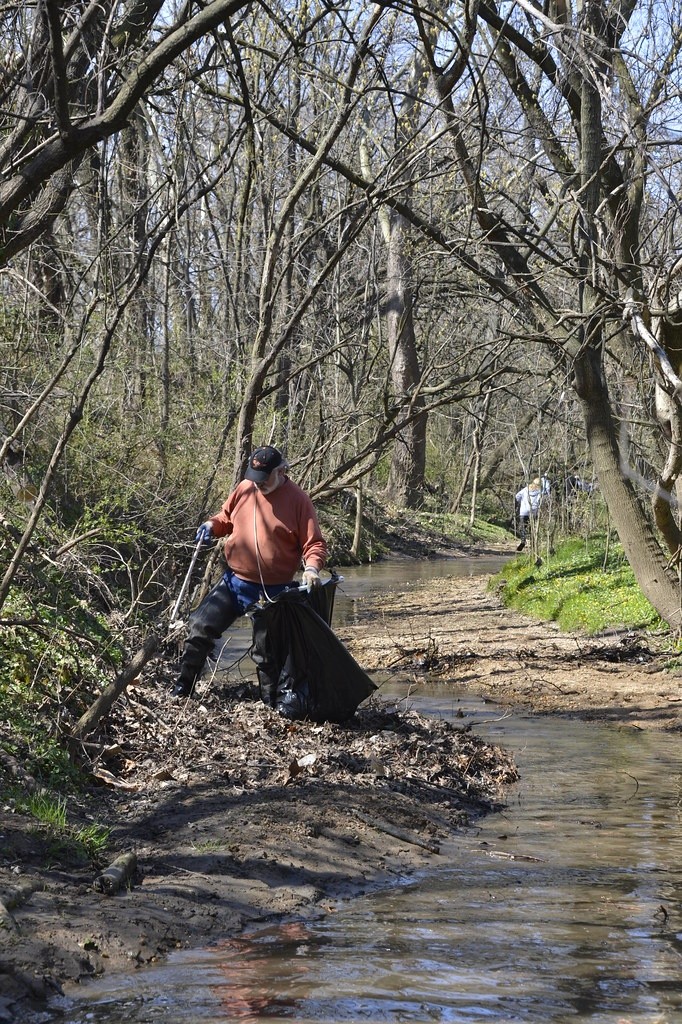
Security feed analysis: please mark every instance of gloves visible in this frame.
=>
[302,570,322,594]
[195,521,214,542]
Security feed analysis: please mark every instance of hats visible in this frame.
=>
[244,446,282,482]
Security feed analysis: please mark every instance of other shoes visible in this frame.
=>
[517,541,525,551]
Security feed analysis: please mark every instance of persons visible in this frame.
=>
[168,445,329,706]
[515,476,544,551]
[539,467,607,510]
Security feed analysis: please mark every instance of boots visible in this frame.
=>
[256,667,278,709]
[170,578,234,698]
